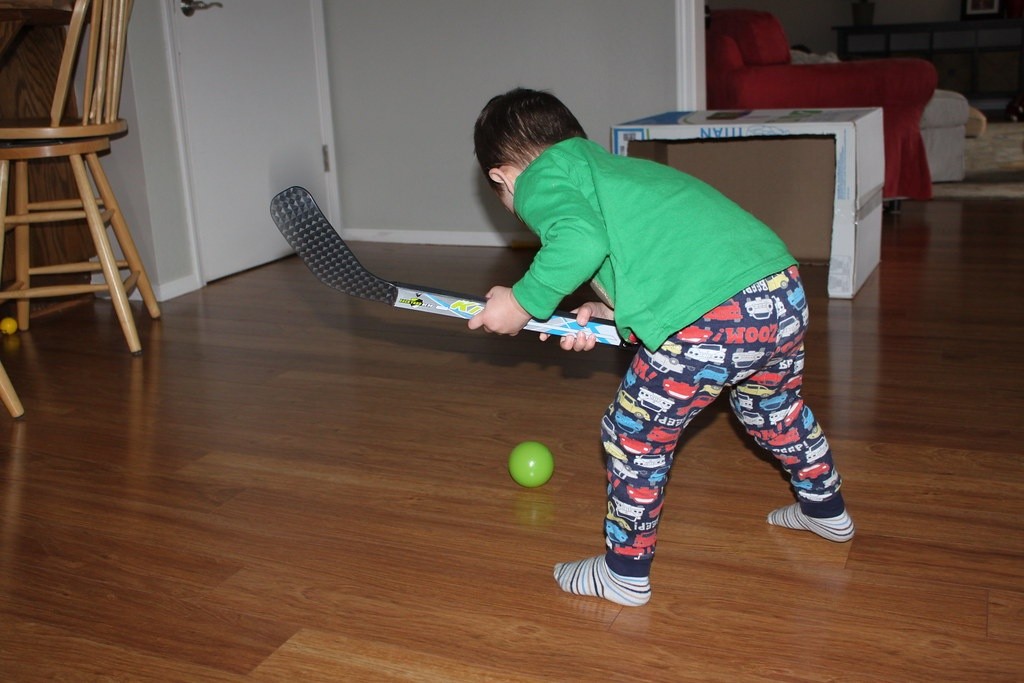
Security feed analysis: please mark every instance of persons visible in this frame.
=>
[467,87,856,608]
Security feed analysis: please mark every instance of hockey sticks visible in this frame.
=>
[268,184,630,348]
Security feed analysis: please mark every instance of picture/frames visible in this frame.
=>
[961,0,1004,20]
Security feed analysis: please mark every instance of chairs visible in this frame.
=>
[0,0,161,353]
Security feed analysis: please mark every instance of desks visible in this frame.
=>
[831,17,1024,99]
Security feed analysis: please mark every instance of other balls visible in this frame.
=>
[508,441,555,488]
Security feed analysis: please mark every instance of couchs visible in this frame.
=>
[701,10,931,216]
